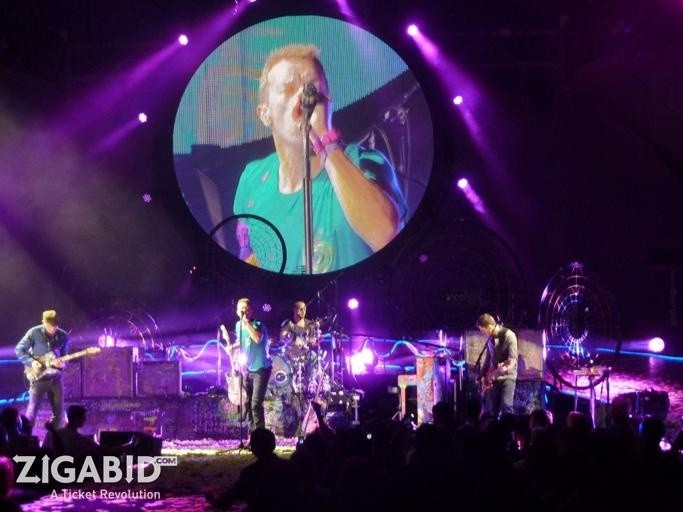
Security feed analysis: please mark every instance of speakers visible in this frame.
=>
[36,347,248,441]
[98,430,160,455]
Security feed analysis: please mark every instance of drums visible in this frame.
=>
[285,345,307,362]
[268,351,291,391]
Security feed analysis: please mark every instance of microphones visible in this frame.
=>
[298,83,318,118]
[238,310,246,316]
[497,314,505,329]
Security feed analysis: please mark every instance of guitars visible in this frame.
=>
[23,346,100,380]
[478,358,510,396]
[219,324,248,405]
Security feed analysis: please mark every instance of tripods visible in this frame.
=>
[215,324,263,456]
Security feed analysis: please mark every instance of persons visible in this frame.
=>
[17,308,73,428]
[224,299,272,447]
[278,299,315,341]
[235,43,408,271]
[4,400,683,512]
[478,309,519,419]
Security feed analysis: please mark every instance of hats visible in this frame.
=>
[42,309,59,328]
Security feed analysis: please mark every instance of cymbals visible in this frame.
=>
[306,316,328,328]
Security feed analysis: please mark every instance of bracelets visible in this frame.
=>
[308,126,346,169]
